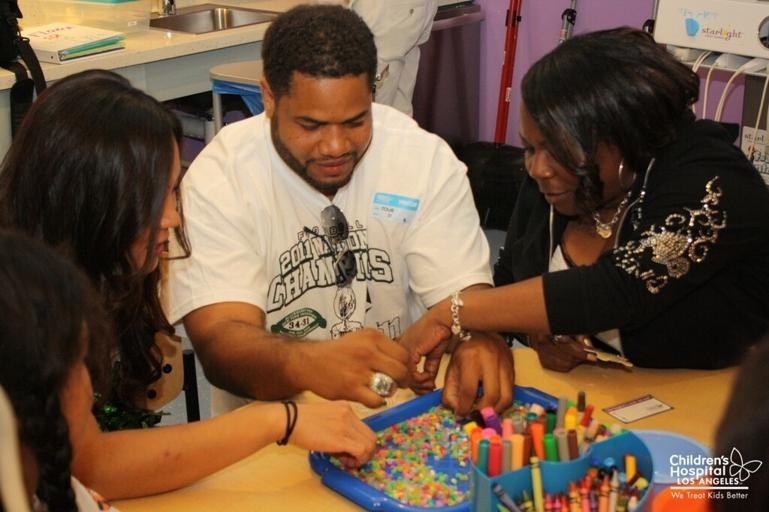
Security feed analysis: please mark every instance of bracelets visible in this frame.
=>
[450,290,471,342]
[278,399,297,445]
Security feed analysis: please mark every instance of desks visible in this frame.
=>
[100,326,769,511]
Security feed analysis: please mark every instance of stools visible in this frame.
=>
[208,56,264,133]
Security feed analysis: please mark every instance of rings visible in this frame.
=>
[372,373,392,395]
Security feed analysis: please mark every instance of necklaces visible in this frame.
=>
[592,191,633,238]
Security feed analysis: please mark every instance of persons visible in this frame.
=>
[709,335,769,511]
[1,71,377,502]
[319,2,439,118]
[0,229,122,511]
[162,7,515,422]
[397,26,768,395]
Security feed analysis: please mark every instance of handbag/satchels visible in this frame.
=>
[1,1,24,72]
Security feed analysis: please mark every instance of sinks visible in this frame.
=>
[149,4,285,36]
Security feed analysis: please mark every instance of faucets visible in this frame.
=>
[162,0,176,14]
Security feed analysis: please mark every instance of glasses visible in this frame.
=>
[304,204,357,288]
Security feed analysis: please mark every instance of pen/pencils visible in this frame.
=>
[455,392,648,512]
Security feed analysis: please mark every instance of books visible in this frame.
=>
[17,23,128,65]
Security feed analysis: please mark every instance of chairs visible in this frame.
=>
[135,336,205,425]
